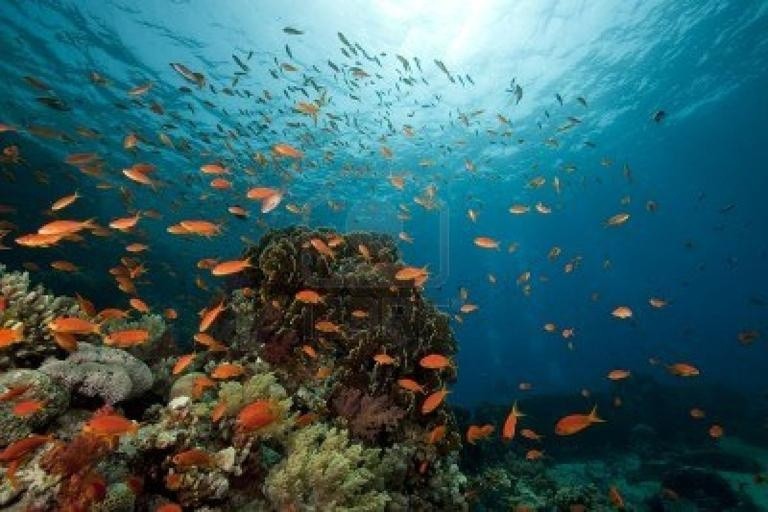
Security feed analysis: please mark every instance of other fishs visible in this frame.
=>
[0,44,767,512]
